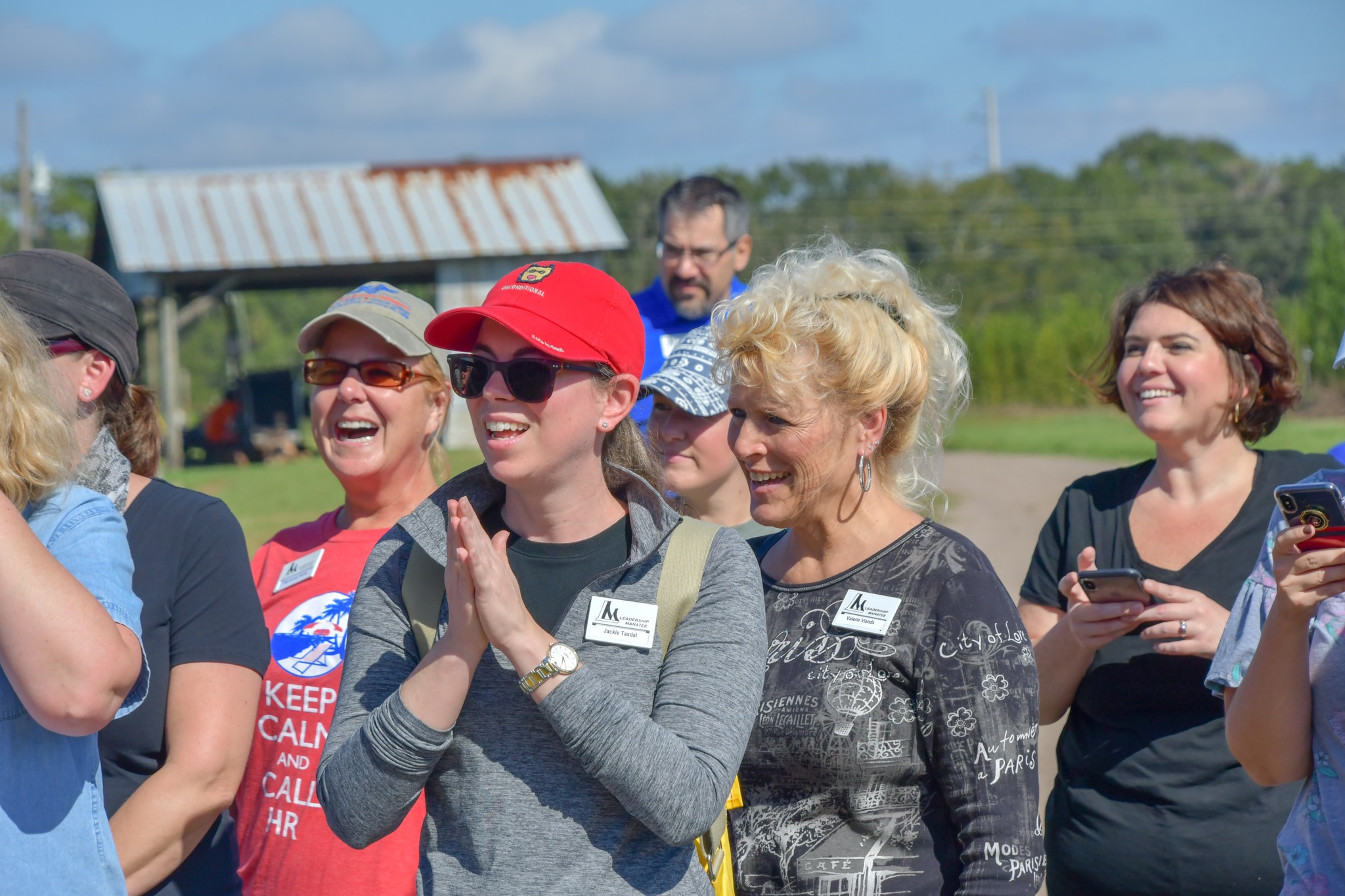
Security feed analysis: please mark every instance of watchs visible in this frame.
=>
[519,642,579,695]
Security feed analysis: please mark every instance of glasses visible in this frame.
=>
[656,240,735,264]
[447,353,609,403]
[304,357,437,388]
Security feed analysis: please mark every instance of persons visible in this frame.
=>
[638,323,776,546]
[631,177,752,441]
[1019,261,1342,896]
[204,390,264,465]
[1,293,149,895]
[0,248,269,896]
[710,234,1047,896]
[237,281,455,895]
[315,260,766,896]
[1204,329,1345,896]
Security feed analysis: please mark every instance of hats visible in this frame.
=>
[421,259,643,384]
[636,324,727,418]
[4,248,140,396]
[299,280,453,387]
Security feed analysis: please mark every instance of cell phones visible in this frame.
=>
[1076,568,1158,636]
[1273,482,1344,556]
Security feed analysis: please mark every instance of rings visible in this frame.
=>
[1180,620,1187,638]
